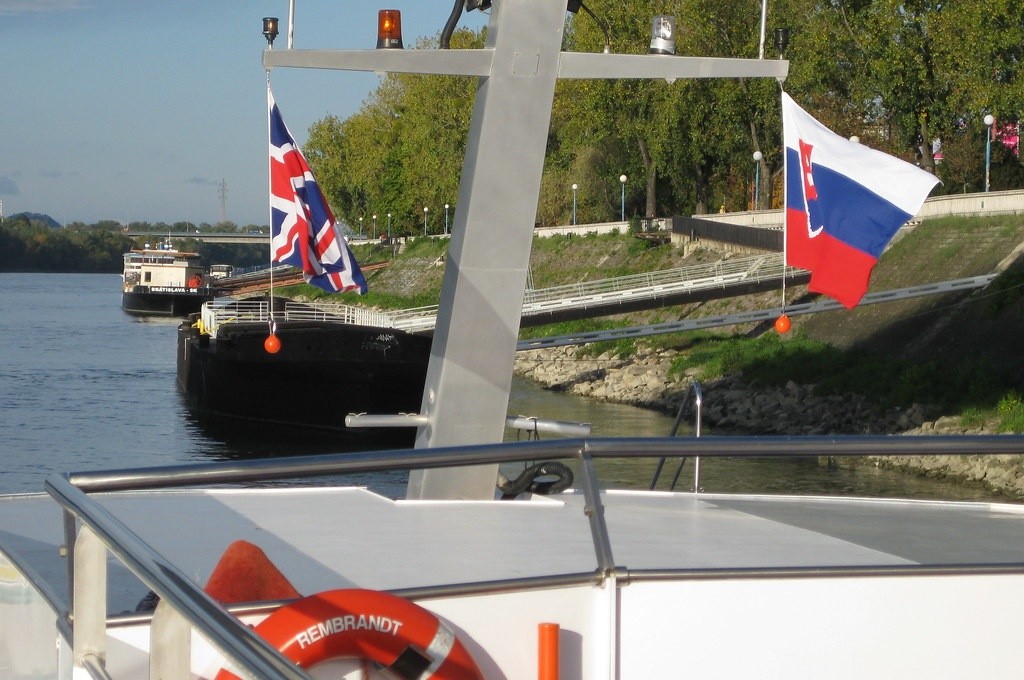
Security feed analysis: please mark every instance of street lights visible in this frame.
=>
[372,214,376,241]
[423,206,428,235]
[359,217,363,242]
[620,174,628,223]
[984,115,994,192]
[444,203,450,234]
[387,213,391,237]
[572,183,578,225]
[752,151,763,210]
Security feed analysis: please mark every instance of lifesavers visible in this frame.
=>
[210,587,486,679]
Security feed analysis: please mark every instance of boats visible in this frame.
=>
[120,230,235,315]
[175,288,434,436]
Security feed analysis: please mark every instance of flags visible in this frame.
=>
[781,91,944,310]
[267,88,368,297]
